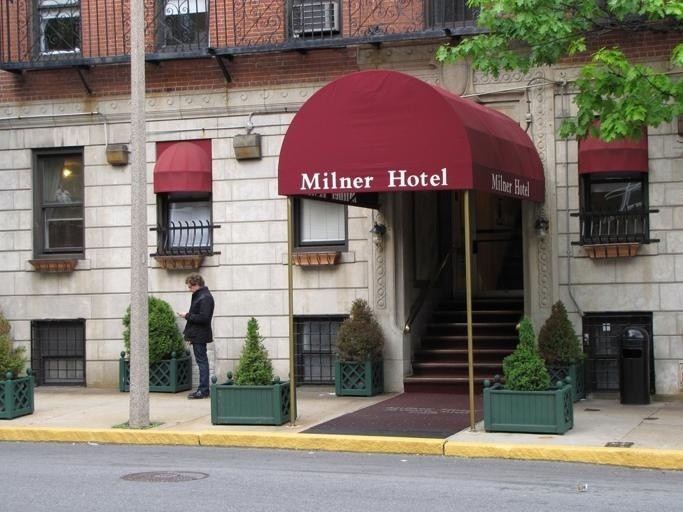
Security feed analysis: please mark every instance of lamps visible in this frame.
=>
[231,134,261,162]
[368,219,387,249]
[104,141,129,166]
[534,216,550,243]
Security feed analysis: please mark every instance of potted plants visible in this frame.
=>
[210,311,295,427]
[537,299,587,403]
[115,291,196,393]
[481,312,576,437]
[332,293,387,398]
[0,312,38,421]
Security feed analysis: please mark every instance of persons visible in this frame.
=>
[178,273,215,398]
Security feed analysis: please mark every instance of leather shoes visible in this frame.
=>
[188,390,208,399]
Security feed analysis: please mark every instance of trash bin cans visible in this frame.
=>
[619,326,651,404]
